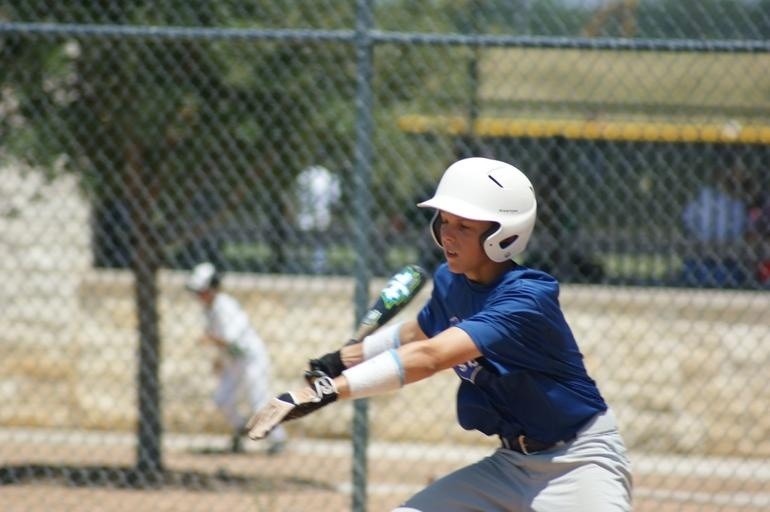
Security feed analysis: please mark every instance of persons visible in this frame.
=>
[243,156,636,512]
[294,149,343,272]
[674,171,746,290]
[183,262,290,455]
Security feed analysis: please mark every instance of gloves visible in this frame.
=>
[303,349,348,385]
[245,376,338,441]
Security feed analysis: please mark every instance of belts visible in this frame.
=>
[501,431,576,457]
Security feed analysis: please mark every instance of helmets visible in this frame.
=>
[416,156,538,264]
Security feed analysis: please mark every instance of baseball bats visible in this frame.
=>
[304,264,427,392]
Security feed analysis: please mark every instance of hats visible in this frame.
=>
[184,263,220,296]
